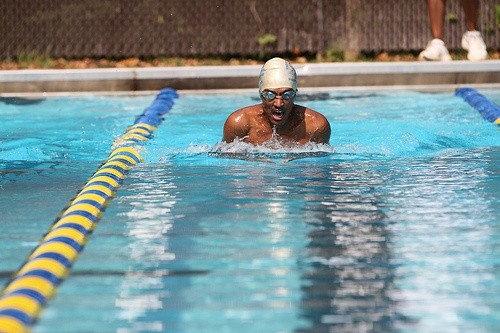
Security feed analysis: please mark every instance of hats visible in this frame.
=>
[259,57,297,94]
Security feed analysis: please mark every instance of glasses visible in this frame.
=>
[260,89,296,100]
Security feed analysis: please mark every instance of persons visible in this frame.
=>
[416,0,490,62]
[223,57,331,150]
